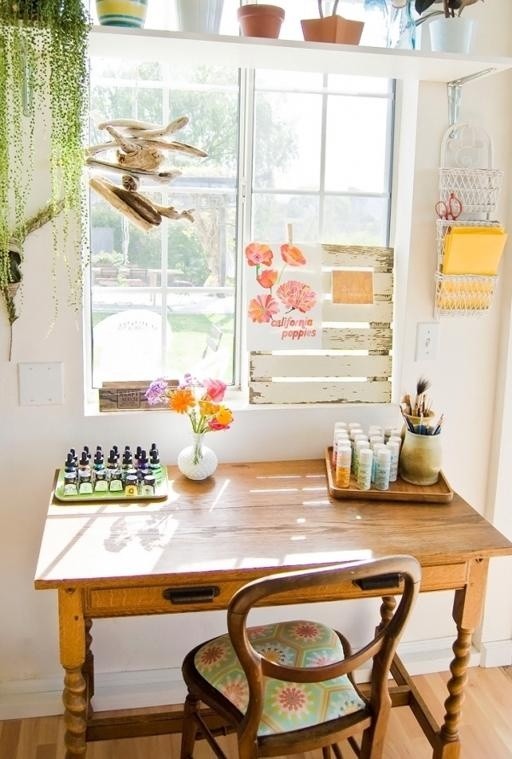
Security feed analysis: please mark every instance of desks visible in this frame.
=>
[33,458,511,759]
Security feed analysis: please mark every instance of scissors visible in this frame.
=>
[436,193,462,220]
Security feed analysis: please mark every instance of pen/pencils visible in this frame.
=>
[421,425,427,434]
[418,408,421,417]
[431,413,444,435]
[417,425,421,433]
[422,394,425,415]
[405,416,415,433]
[427,427,434,435]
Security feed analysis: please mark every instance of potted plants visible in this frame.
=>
[415,0,478,52]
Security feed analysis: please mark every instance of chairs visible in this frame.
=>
[180,554,422,759]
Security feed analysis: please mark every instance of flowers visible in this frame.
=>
[144,373,240,464]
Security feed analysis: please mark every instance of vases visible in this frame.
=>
[96,0,148,28]
[177,434,218,481]
[236,4,285,39]
[300,15,365,45]
[175,0,224,34]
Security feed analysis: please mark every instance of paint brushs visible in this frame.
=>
[400,394,411,415]
[413,378,430,416]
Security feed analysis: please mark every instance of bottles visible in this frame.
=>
[356,449,374,491]
[352,434,369,475]
[372,443,385,482]
[126,469,138,477]
[338,439,351,451]
[64,452,77,477]
[112,445,120,463]
[331,429,347,466]
[138,450,149,479]
[77,451,91,483]
[93,445,105,458]
[122,450,134,479]
[109,470,125,492]
[386,441,400,482]
[141,469,153,479]
[390,429,401,437]
[149,443,161,465]
[69,448,78,468]
[347,421,362,434]
[337,434,350,445]
[355,441,371,482]
[141,475,157,496]
[123,445,134,457]
[336,446,352,488]
[334,421,348,429]
[389,437,402,447]
[369,436,386,447]
[124,475,139,496]
[63,474,78,495]
[78,472,94,494]
[369,424,383,431]
[134,446,142,468]
[107,449,119,479]
[149,451,163,483]
[374,449,391,491]
[92,451,106,480]
[92,471,109,492]
[384,427,398,445]
[83,445,92,464]
[368,430,383,441]
[350,428,363,446]
